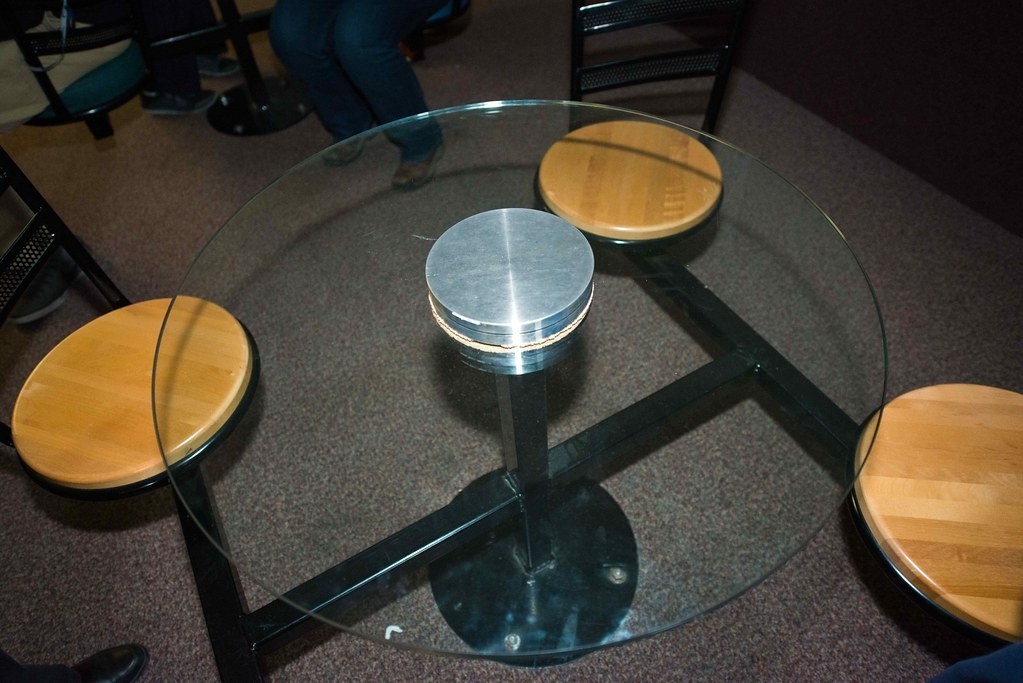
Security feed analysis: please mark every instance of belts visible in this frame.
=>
[70,644,150,683]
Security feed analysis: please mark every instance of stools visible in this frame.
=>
[853,381,1023,640]
[538,120,722,242]
[401,0,471,63]
[24,27,145,141]
[12,297,253,489]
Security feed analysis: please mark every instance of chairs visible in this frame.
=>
[571,0,749,141]
[0,143,132,459]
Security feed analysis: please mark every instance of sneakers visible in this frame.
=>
[391,144,445,190]
[198,56,239,77]
[322,138,366,165]
[140,90,217,115]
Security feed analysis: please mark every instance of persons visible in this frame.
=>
[64,0,242,116]
[0,642,149,683]
[268,0,451,191]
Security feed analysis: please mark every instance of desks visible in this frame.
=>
[150,99,894,667]
